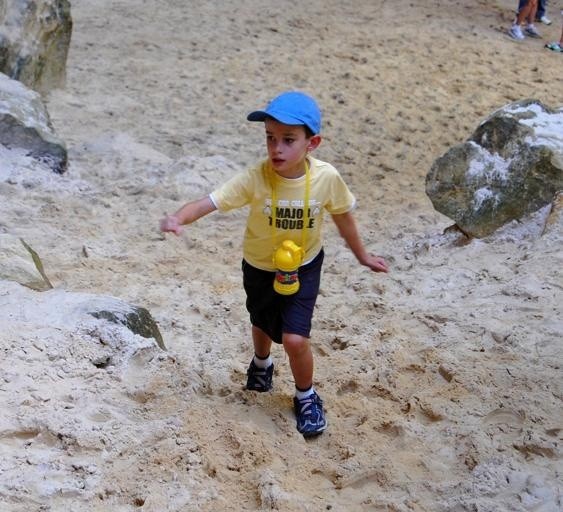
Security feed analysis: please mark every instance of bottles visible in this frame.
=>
[272,239,305,297]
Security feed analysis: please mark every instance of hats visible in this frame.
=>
[247,90,322,135]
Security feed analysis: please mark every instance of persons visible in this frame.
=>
[506,1,543,43]
[159,90,390,439]
[545,28,562,53]
[517,0,553,29]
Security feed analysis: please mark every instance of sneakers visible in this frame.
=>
[524,27,543,39]
[536,16,551,25]
[545,42,563,53]
[293,390,327,436]
[246,358,274,391]
[507,27,527,43]
